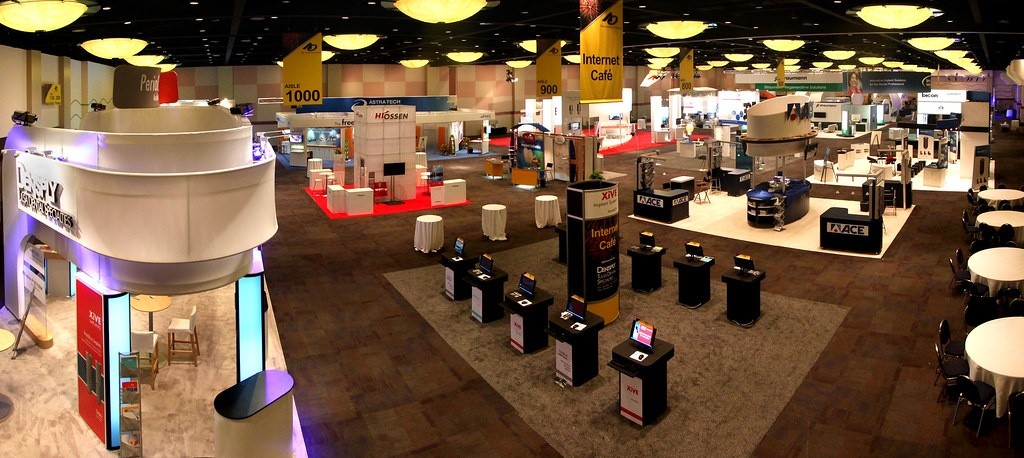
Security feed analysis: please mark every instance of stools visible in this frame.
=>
[168,306,200,367]
[131,331,160,391]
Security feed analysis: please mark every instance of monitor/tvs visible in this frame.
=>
[565,290,587,320]
[479,254,494,275]
[569,122,580,130]
[518,273,536,297]
[629,320,656,349]
[734,254,755,270]
[384,162,405,176]
[639,232,655,247]
[453,236,465,256]
[684,242,704,256]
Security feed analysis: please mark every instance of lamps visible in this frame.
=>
[0,0,983,78]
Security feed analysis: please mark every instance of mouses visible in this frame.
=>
[522,301,527,304]
[639,355,644,359]
[573,325,579,329]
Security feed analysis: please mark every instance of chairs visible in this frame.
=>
[935,184,1024,449]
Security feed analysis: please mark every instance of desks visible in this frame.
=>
[977,189,1024,210]
[130,294,172,365]
[964,317,1024,417]
[968,247,1024,297]
[308,125,925,451]
[0,328,16,419]
[975,211,1024,245]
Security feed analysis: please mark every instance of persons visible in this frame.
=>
[847,72,862,94]
